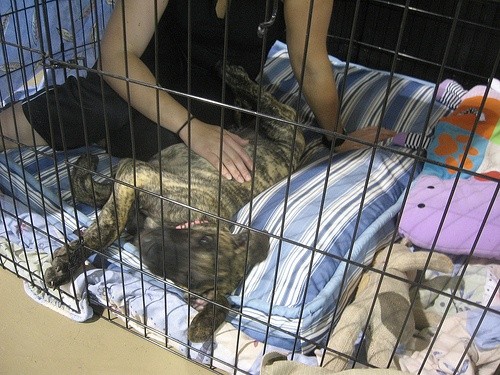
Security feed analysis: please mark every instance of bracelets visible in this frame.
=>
[175,116,196,136]
[322,127,348,148]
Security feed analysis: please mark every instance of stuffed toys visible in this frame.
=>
[259,351,406,375]
[385,80,500,264]
[314,239,458,375]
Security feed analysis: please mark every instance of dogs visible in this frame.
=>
[43,59,305,341]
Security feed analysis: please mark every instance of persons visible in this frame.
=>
[0,0,398,184]
